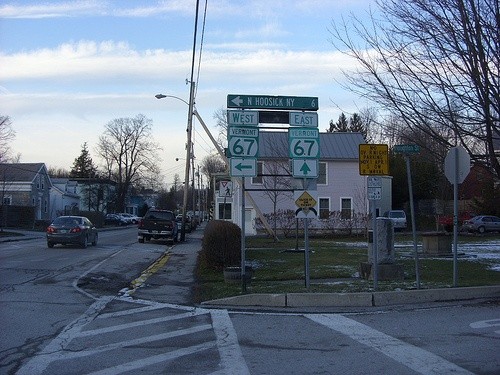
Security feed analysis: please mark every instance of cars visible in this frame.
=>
[106,212,143,225]
[46,215,98,248]
[463,215,500,234]
[175,211,208,233]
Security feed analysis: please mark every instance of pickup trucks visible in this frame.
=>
[138,209,179,245]
[436,208,481,233]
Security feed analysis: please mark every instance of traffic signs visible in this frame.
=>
[226,94,318,111]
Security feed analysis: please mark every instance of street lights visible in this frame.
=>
[154,93,194,241]
[175,157,196,232]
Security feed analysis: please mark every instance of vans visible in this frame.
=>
[383,210,407,231]
